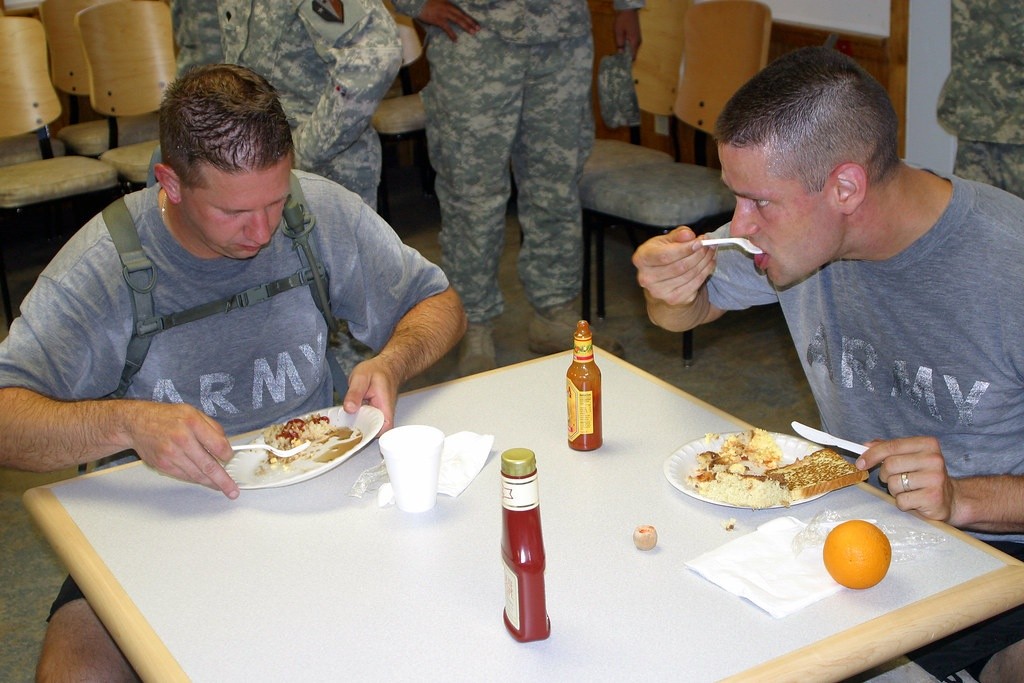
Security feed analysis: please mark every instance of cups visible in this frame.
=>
[379,424,447,514]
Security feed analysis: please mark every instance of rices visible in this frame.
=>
[263,414,335,463]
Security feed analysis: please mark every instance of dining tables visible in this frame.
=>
[24,342,1023,683]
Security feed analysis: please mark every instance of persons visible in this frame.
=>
[168,0,404,213]
[0,64,468,683]
[629,45,1024,683]
[389,1,644,379]
[937,0,1024,204]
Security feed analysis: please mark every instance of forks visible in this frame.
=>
[225,440,310,459]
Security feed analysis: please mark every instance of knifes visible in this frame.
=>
[790,421,886,464]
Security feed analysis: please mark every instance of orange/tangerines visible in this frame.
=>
[823,520,890,589]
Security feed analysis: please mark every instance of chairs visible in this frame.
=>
[0,0,773,370]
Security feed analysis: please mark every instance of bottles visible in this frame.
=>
[566,320,604,451]
[500,448,553,642]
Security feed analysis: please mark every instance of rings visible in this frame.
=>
[901,472,910,492]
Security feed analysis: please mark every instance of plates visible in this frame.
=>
[664,431,839,509]
[221,403,385,491]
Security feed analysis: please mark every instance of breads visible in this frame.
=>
[764,447,871,501]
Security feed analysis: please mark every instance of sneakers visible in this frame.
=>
[528,312,624,358]
[458,323,496,378]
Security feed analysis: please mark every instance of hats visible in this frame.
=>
[598,40,642,127]
